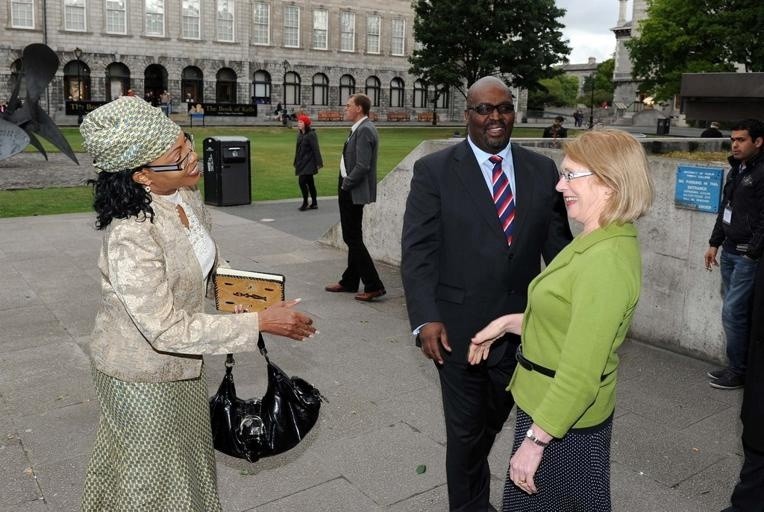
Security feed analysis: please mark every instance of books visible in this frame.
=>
[213,266,285,312]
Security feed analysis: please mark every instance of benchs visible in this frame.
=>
[317,111,440,122]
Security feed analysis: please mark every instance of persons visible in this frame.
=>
[293,115,323,210]
[467,127,656,512]
[704,119,763,391]
[701,121,723,138]
[273,102,296,124]
[579,111,584,127]
[325,93,387,301]
[126,88,194,104]
[400,75,574,512]
[573,110,580,127]
[542,116,567,149]
[77,95,321,512]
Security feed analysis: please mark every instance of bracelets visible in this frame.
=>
[526,428,549,448]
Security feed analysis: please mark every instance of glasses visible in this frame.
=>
[467,103,514,115]
[143,132,195,171]
[558,170,593,183]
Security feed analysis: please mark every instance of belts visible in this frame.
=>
[516,344,615,381]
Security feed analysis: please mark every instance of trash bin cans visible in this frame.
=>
[203,136,251,207]
[657,118,670,136]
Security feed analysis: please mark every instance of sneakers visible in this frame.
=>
[707,370,744,390]
[299,204,318,210]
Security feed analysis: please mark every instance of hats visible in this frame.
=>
[299,114,311,126]
[711,122,720,129]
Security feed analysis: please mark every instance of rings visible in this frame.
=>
[518,480,526,484]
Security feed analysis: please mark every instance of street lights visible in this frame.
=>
[74,47,83,123]
[282,59,290,124]
[589,72,597,130]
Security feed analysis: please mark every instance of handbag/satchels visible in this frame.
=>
[208,362,322,463]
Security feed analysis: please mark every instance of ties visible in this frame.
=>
[488,155,516,247]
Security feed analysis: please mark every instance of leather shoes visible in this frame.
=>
[325,283,358,293]
[355,288,385,301]
